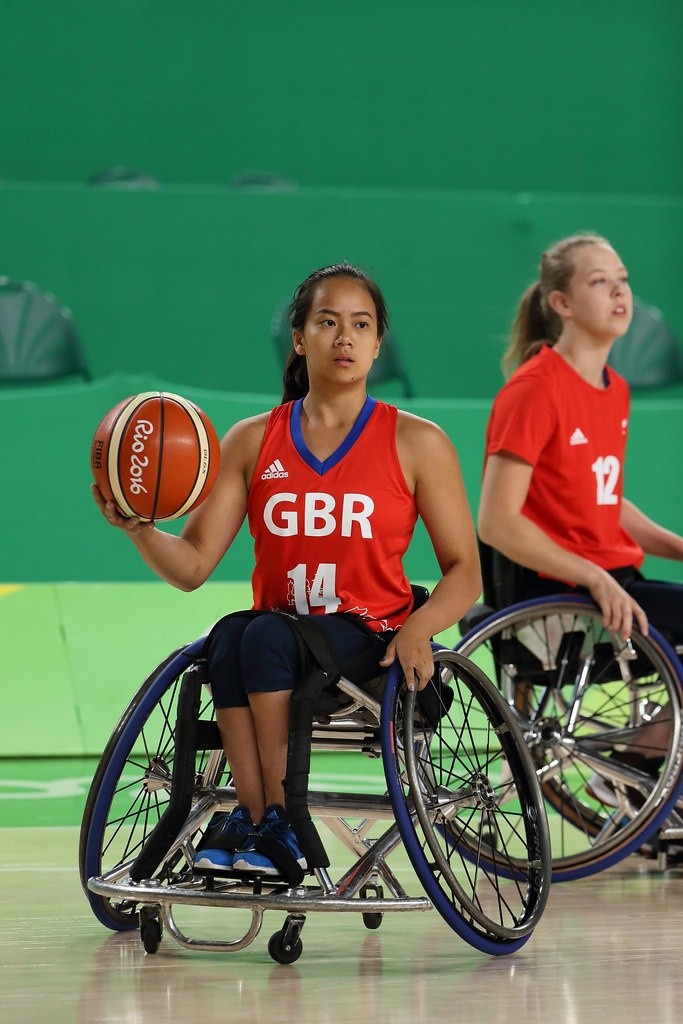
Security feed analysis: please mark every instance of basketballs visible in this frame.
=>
[88,390,223,525]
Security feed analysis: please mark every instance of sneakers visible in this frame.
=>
[193,805,265,871]
[584,750,683,827]
[232,804,308,888]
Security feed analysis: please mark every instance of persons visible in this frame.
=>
[90,263,485,875]
[474,233,683,768]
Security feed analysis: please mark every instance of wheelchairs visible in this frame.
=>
[79,584,555,967]
[414,590,683,882]
